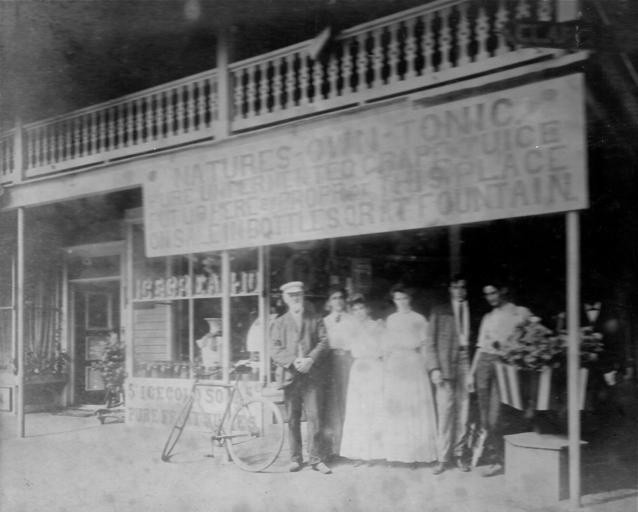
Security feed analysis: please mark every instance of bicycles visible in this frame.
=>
[154,358,286,474]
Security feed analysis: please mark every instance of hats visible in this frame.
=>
[327,284,348,299]
[280,281,304,293]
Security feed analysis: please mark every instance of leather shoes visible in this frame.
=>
[432,462,470,475]
[289,462,331,474]
[483,463,504,477]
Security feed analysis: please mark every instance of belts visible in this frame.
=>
[459,345,468,351]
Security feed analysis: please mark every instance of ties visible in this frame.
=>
[459,303,464,335]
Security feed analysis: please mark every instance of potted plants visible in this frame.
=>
[475,310,605,413]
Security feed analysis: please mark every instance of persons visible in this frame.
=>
[269,274,536,477]
